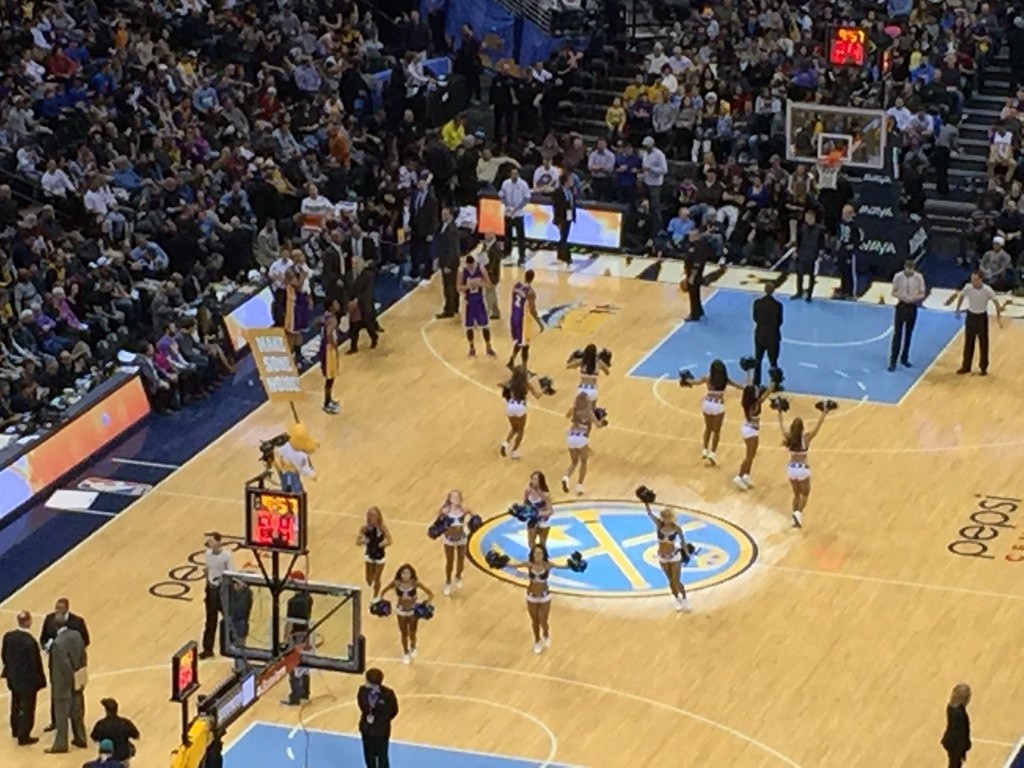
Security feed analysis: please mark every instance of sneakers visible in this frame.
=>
[734,474,754,491]
[534,635,551,653]
[675,599,691,612]
[702,449,718,465]
[444,575,463,595]
[322,400,340,414]
[500,443,521,459]
[791,511,802,528]
[402,647,417,666]
[562,476,585,495]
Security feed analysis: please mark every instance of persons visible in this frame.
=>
[0,0,1024,768]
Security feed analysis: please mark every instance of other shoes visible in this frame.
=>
[13,734,40,746]
[507,363,538,376]
[888,361,911,372]
[791,293,811,302]
[200,650,214,659]
[43,723,57,732]
[72,740,86,748]
[45,747,67,754]
[347,340,380,357]
[956,368,986,375]
[468,348,495,358]
[281,691,310,705]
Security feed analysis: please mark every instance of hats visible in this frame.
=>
[993,237,1005,246]
[101,698,118,712]
[101,739,113,751]
